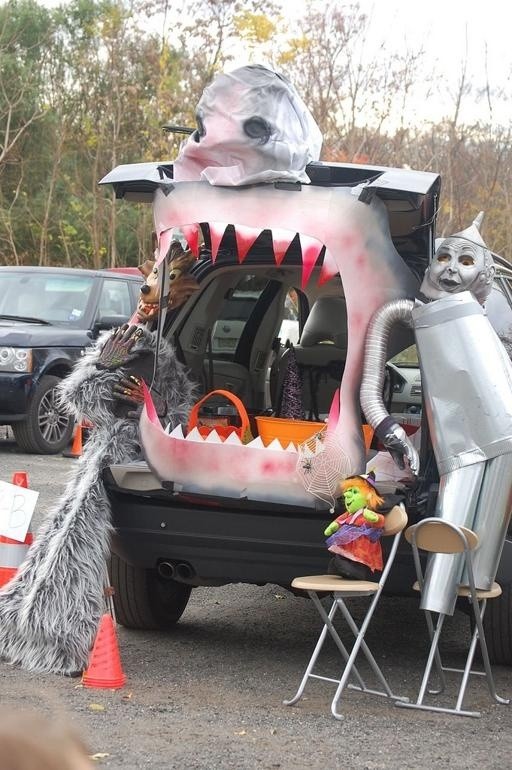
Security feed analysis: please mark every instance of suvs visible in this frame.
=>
[84,160,511,667]
[0,265,145,456]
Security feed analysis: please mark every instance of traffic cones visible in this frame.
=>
[82,614,126,689]
[0,471,35,589]
[61,423,83,458]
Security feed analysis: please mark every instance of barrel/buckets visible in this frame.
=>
[255,416,373,455]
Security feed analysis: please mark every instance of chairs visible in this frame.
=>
[264,291,349,419]
[283,505,410,725]
[390,513,511,724]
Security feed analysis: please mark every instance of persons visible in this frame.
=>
[323,470,385,580]
[420,223,495,307]
